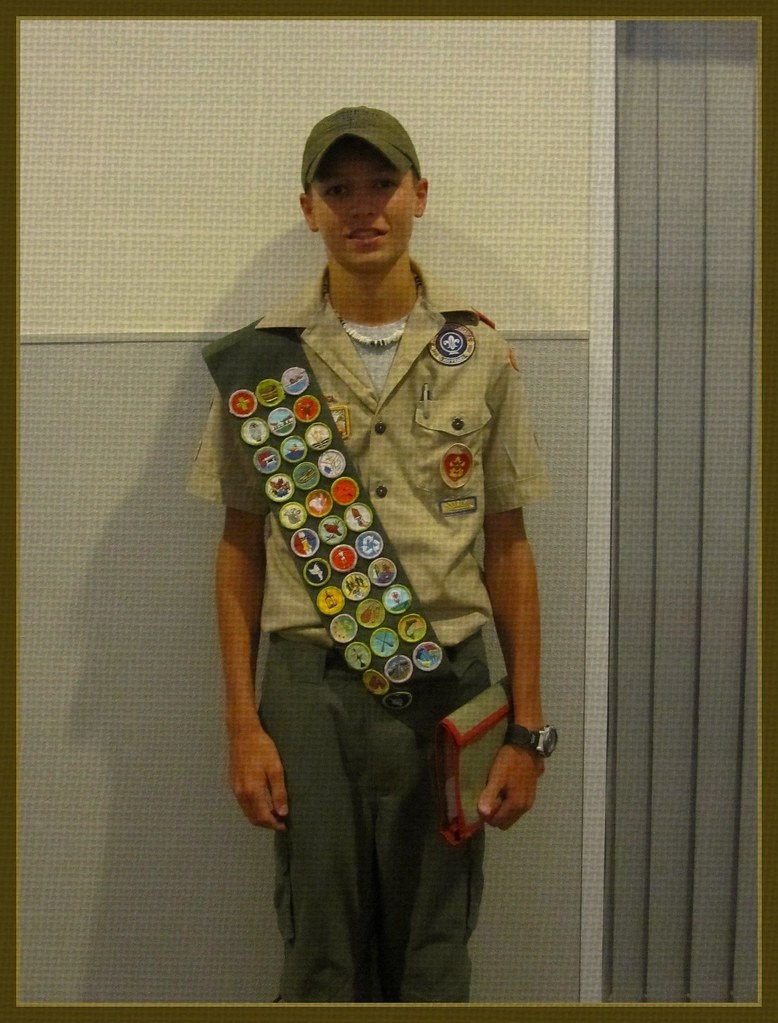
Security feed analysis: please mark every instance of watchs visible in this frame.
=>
[504,724,559,756]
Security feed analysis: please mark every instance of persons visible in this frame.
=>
[185,108,546,1005]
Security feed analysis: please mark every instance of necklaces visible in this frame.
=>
[323,268,422,346]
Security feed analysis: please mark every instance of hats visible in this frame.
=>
[302,105,421,198]
